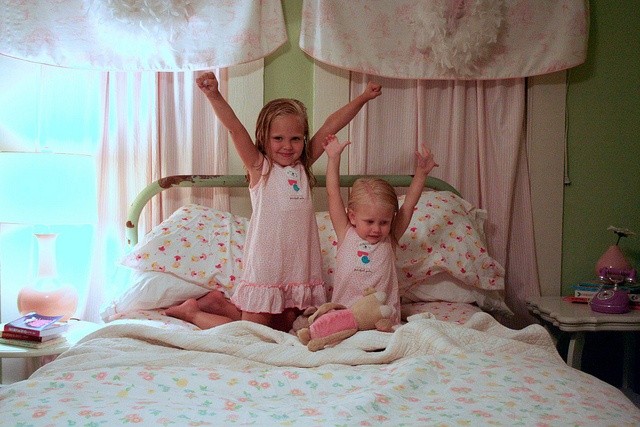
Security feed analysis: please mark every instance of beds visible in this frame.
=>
[0,174,640,427]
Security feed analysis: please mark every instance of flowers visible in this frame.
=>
[606,224,637,246]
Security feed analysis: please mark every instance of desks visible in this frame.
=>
[0,318,99,385]
[525,292,640,371]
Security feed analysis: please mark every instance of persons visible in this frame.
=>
[322,133,439,326]
[162,70,382,332]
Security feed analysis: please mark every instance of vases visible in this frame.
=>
[17,232,77,323]
[594,243,631,279]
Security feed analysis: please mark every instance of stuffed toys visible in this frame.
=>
[297,288,392,353]
[292,307,316,333]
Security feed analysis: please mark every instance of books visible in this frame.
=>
[561,280,640,303]
[4,312,68,337]
[0,331,62,342]
[0,338,66,349]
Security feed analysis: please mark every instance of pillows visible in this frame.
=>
[315,190,507,293]
[120,203,250,298]
[100,268,212,322]
[400,272,514,323]
